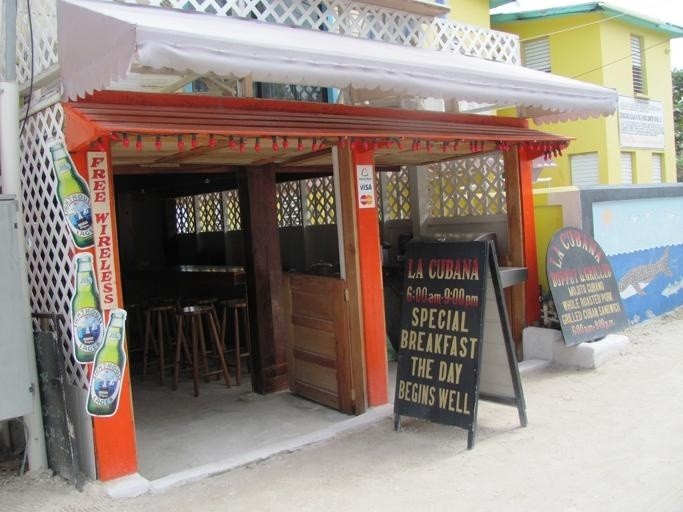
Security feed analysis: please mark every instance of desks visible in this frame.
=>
[124,263,248,355]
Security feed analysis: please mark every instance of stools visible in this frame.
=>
[126,293,254,399]
